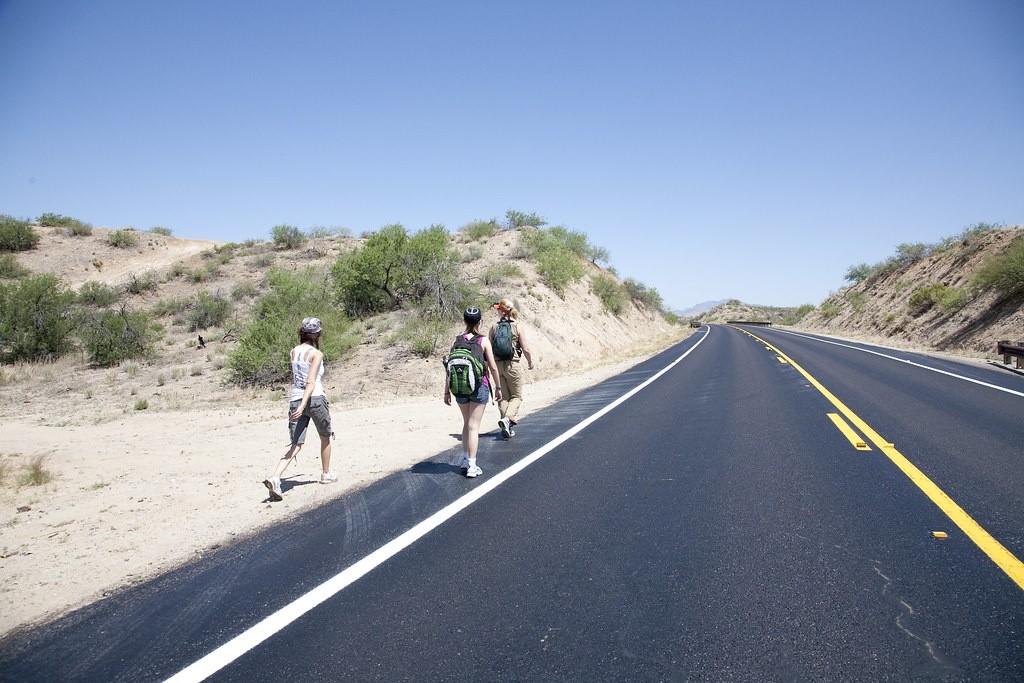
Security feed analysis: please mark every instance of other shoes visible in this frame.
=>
[498,417,510,441]
[510,430,515,436]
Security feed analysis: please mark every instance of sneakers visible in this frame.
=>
[466,466,483,478]
[459,452,470,469]
[321,473,338,484]
[264,476,282,501]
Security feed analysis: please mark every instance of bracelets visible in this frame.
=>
[496,386,501,390]
[445,392,450,395]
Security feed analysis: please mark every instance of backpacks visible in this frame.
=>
[492,319,523,361]
[446,334,486,399]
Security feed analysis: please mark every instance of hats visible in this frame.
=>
[301,317,322,333]
[494,299,514,315]
[464,308,481,325]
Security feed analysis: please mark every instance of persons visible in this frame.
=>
[488,298,534,442]
[442,306,503,478]
[264,318,338,502]
